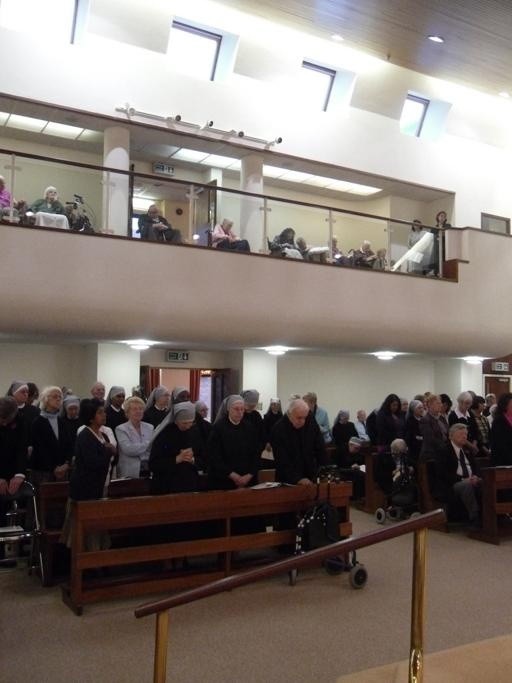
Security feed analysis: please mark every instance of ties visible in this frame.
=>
[460,448,469,477]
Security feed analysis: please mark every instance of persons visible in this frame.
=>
[0,177,95,232]
[406,210,451,277]
[333,389,510,526]
[1,382,349,563]
[267,228,387,269]
[138,203,251,252]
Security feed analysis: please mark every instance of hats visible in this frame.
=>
[349,436,363,447]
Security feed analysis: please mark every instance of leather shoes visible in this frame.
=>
[91,554,248,581]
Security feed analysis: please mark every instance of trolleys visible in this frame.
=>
[371,449,418,526]
[287,464,368,588]
[65,194,93,232]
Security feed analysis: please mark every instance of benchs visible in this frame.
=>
[0,422,512,614]
